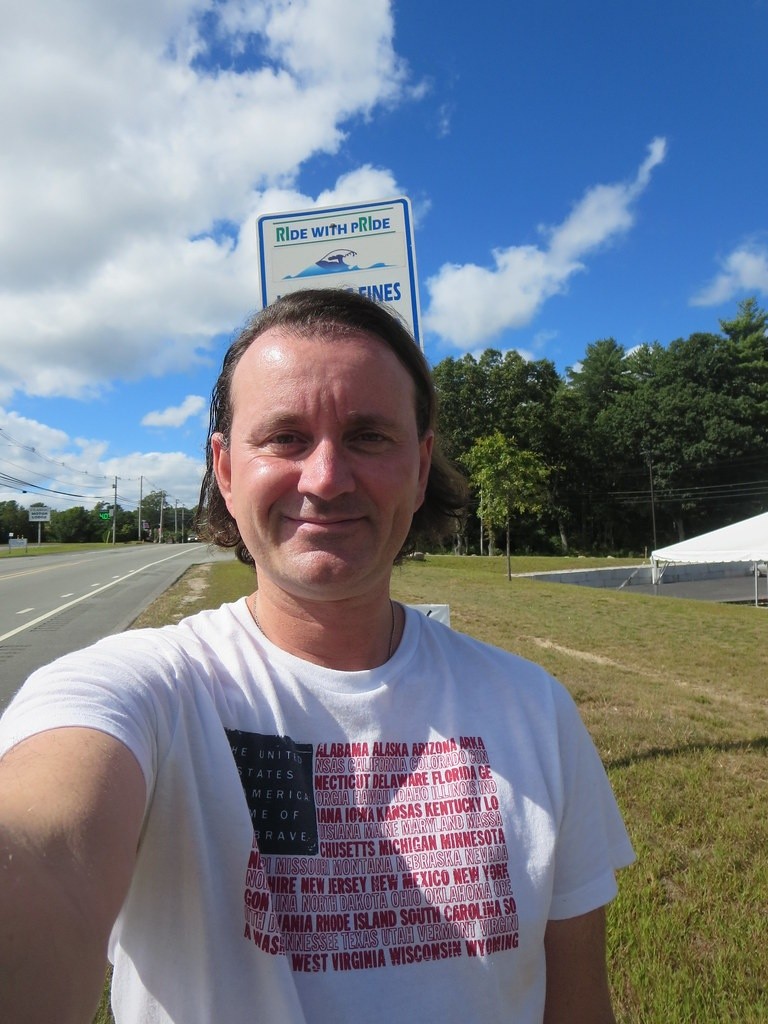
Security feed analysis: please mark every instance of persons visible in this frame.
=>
[0,291,639,1024]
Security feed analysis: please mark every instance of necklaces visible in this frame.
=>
[254,591,395,659]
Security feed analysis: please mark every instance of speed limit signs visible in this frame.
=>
[100,512,109,520]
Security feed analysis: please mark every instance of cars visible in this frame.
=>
[188,533,202,542]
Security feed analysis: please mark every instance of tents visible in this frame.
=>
[651,512,768,607]
